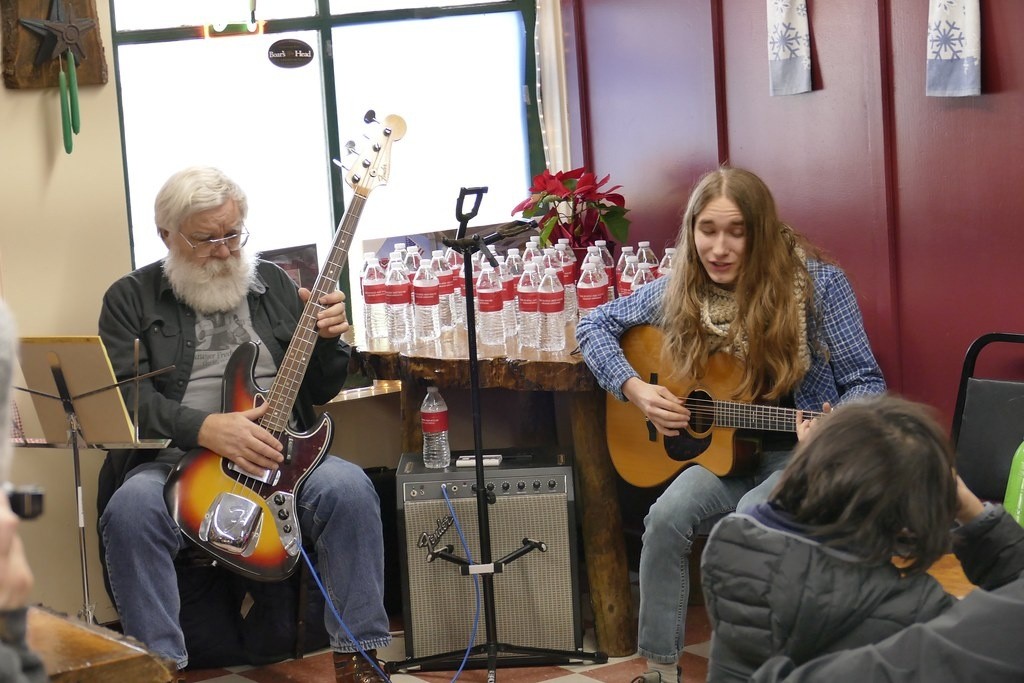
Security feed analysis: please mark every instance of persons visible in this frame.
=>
[96,166,392,683]
[751,467,1024,683]
[574,167,888,683]
[0,299,50,683]
[699,398,963,683]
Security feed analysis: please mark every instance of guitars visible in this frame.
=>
[162,107,407,580]
[605,320,830,488]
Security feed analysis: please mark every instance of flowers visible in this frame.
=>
[511,165,632,248]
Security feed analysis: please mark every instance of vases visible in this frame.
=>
[572,242,617,277]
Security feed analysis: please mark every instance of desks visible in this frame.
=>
[372,347,637,657]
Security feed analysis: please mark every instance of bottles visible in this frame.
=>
[420,386,451,468]
[361,234,678,350]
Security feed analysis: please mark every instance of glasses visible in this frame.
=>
[173,220,250,258]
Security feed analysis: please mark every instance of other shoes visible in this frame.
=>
[333,649,390,683]
[631,665,682,683]
